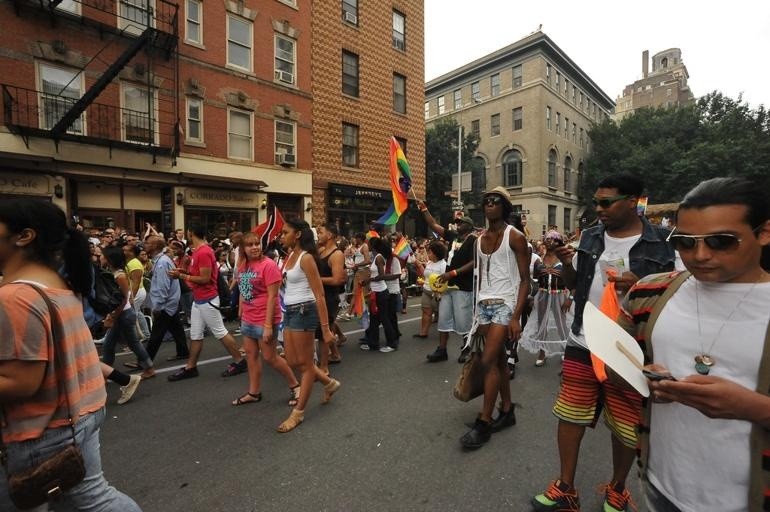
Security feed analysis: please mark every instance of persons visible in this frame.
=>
[1,194,142,511]
[412,186,543,448]
[58,216,417,433]
[529,173,769,510]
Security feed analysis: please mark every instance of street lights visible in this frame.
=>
[457,97,482,212]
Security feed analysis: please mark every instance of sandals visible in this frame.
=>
[277,407,305,432]
[288,383,301,406]
[320,378,341,406]
[232,391,263,405]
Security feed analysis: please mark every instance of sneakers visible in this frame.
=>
[358,332,428,352]
[336,336,347,347]
[602,477,629,512]
[166,366,199,381]
[458,345,471,363]
[427,347,448,362]
[221,357,248,376]
[117,374,142,405]
[529,479,582,511]
[327,355,342,363]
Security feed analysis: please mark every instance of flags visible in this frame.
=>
[375,136,414,227]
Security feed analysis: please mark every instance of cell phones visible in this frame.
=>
[554,238,565,248]
[642,369,667,381]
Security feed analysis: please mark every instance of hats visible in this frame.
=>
[485,185,513,213]
[455,215,473,227]
[97,243,126,269]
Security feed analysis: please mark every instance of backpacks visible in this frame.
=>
[87,258,125,316]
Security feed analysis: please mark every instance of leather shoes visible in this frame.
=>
[123,359,144,370]
[535,356,547,366]
[460,412,492,449]
[489,404,517,431]
[167,355,189,362]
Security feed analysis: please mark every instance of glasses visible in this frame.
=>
[482,196,503,204]
[663,225,764,252]
[590,195,633,208]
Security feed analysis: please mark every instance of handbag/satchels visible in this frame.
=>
[7,443,87,510]
[453,332,500,403]
